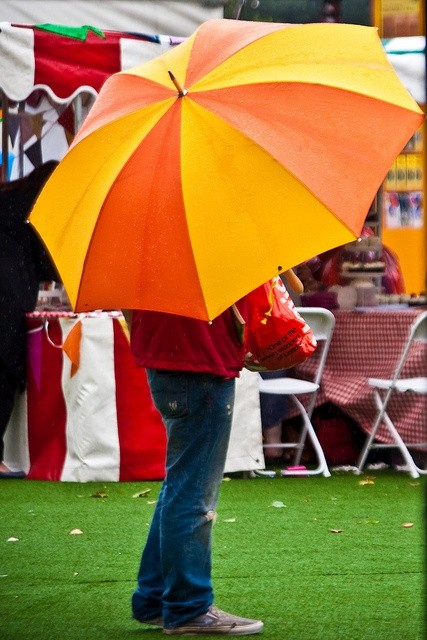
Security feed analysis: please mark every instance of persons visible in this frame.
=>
[258,369,302,463]
[120,307,266,636]
[0,89,69,480]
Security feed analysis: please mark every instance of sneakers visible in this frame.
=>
[163,605,263,635]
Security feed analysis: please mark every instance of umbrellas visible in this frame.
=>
[26,16,424,326]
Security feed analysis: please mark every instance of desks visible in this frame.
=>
[1,306,267,480]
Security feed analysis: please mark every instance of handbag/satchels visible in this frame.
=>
[234,276,318,372]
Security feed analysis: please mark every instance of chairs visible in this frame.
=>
[254,307,335,477]
[354,309,427,479]
